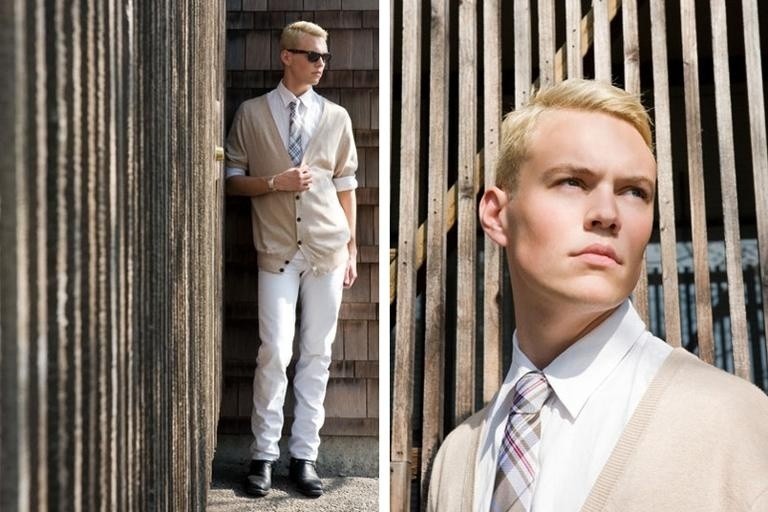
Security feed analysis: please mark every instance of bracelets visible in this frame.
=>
[266,176,276,192]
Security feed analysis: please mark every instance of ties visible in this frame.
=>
[489,372,552,512]
[288,98,303,167]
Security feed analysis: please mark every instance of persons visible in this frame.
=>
[423,76,768,512]
[225,20,358,497]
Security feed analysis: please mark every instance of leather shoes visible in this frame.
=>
[245,460,272,497]
[289,457,323,495]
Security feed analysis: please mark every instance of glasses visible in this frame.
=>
[288,48,331,63]
